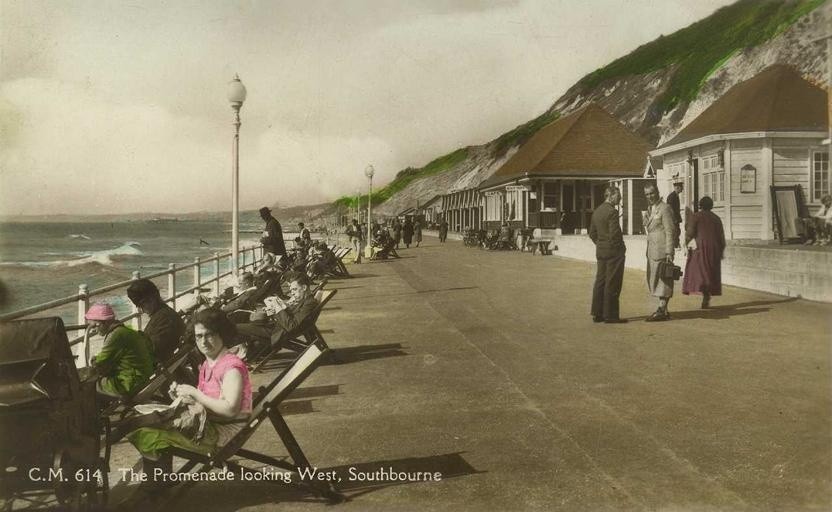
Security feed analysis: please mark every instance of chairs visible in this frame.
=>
[1,244,352,512]
[459,224,555,256]
[375,234,401,260]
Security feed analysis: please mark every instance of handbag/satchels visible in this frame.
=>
[657,262,683,280]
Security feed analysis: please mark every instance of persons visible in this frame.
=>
[588,187,630,323]
[491,222,512,249]
[812,195,831,246]
[684,196,725,309]
[119,308,255,510]
[215,270,260,309]
[666,180,683,250]
[251,204,424,278]
[438,217,450,243]
[640,183,678,321]
[80,303,155,405]
[127,278,186,359]
[228,273,317,361]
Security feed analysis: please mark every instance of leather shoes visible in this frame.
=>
[647,309,673,321]
[593,315,627,323]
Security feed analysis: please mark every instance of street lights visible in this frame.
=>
[333,188,361,235]
[365,165,375,258]
[227,72,246,289]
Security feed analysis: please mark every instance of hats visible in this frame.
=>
[86,305,115,321]
[127,279,156,305]
